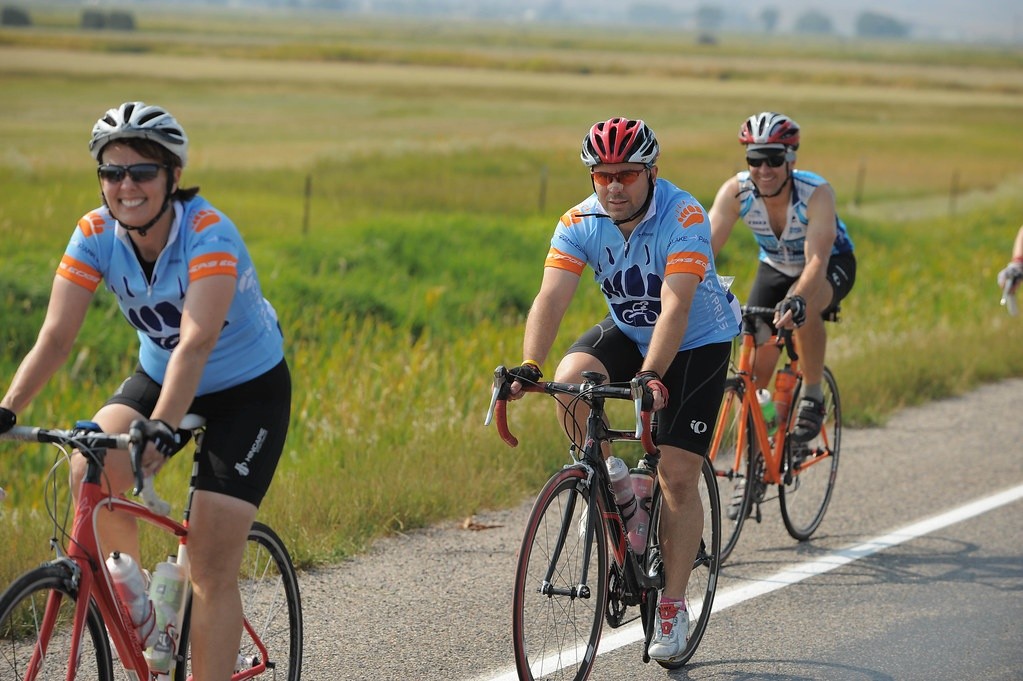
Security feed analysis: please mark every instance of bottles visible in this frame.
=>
[606,456,639,531]
[755,389,778,434]
[627,459,654,555]
[773,363,795,424]
[142,555,185,672]
[105,551,160,647]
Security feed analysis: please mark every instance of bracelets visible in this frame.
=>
[521,360,541,371]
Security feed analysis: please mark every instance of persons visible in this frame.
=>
[491,116,740,660]
[705,111,857,520]
[998,224,1023,295]
[0,101,292,681]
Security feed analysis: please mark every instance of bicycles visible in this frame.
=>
[482,364,722,681]
[1,415,306,681]
[698,298,842,571]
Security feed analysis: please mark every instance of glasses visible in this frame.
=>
[590,166,650,186]
[746,152,790,168]
[98,163,170,181]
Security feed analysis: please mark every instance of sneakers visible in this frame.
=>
[578,504,597,541]
[727,479,752,520]
[647,601,690,660]
[793,396,825,441]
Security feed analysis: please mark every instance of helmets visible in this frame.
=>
[579,116,659,168]
[738,111,800,152]
[88,101,187,168]
